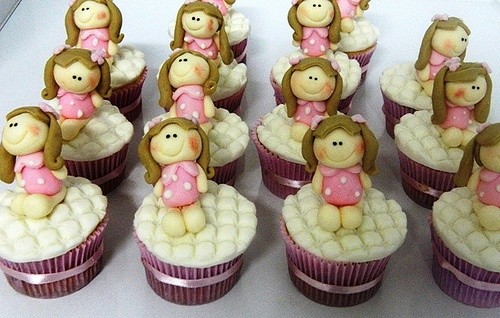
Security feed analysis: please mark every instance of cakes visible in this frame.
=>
[143,48,250,187]
[269,0,379,116]
[252,56,344,199]
[168,0,250,114]
[429,123,500,309]
[132,115,258,305]
[394,56,492,209]
[40,44,134,188]
[0,102,109,299]
[63,0,148,114]
[379,14,471,140]
[279,113,407,307]
